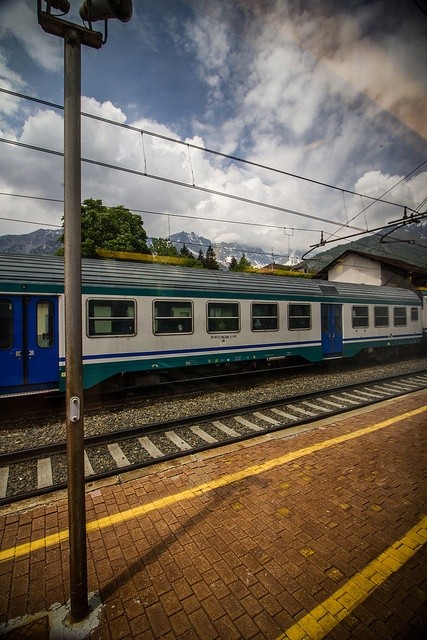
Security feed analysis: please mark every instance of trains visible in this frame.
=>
[0,252,425,388]
